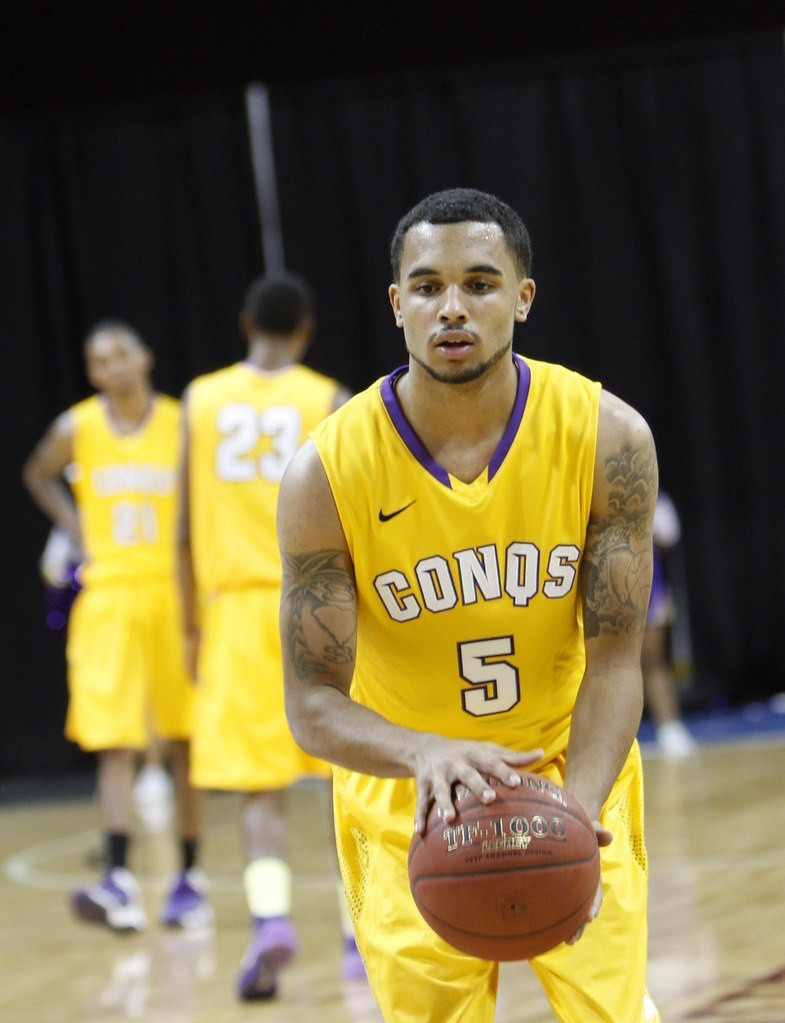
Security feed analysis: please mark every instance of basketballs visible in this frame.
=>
[408,769,601,961]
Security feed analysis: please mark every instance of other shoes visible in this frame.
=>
[656,720,696,758]
[139,768,173,828]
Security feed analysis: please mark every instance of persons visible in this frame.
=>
[177,268,368,1001]
[643,494,698,757]
[23,323,219,933]
[275,187,658,1022]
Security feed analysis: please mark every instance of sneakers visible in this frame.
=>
[160,876,214,932]
[77,876,142,932]
[237,917,297,1001]
[340,936,364,975]
